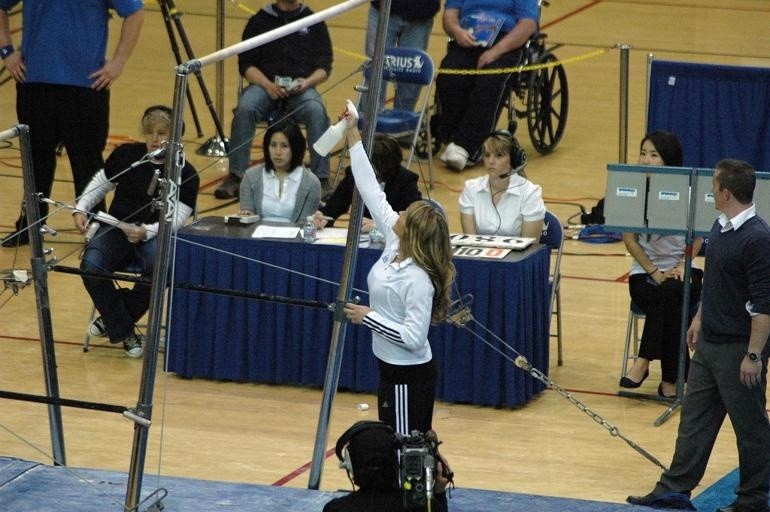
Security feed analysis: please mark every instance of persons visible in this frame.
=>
[358,1,442,109]
[430,0,544,171]
[625,158,770,511]
[456,130,546,246]
[341,109,458,437]
[214,0,333,200]
[0,1,145,246]
[306,132,422,230]
[322,421,454,511]
[238,118,321,228]
[619,127,704,403]
[72,106,200,359]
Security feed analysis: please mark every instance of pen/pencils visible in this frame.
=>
[306,216,334,221]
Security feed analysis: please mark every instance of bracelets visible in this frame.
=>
[1,43,14,60]
[649,267,659,277]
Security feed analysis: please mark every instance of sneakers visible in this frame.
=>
[123,333,142,358]
[215,175,239,199]
[2,231,29,247]
[440,142,468,170]
[89,316,109,337]
[320,179,334,203]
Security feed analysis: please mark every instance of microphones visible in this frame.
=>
[499,165,525,178]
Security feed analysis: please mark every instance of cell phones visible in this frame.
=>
[275,75,292,90]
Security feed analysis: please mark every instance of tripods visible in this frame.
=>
[619,237,693,426]
[55,0,229,156]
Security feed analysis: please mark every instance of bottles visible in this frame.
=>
[304,216,317,243]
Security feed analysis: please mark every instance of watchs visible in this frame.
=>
[742,352,762,363]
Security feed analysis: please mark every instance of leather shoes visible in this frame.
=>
[717,504,743,512]
[658,382,676,398]
[620,369,649,388]
[626,495,650,505]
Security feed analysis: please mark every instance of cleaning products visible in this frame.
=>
[312,99,360,158]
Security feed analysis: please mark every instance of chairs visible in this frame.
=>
[332,48,436,193]
[539,211,564,366]
[621,269,702,378]
[83,204,197,353]
[232,72,331,163]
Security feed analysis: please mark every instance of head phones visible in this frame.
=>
[490,128,527,171]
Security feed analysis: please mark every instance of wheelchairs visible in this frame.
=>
[413,1,569,158]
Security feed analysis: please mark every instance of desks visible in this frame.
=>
[164,215,552,409]
